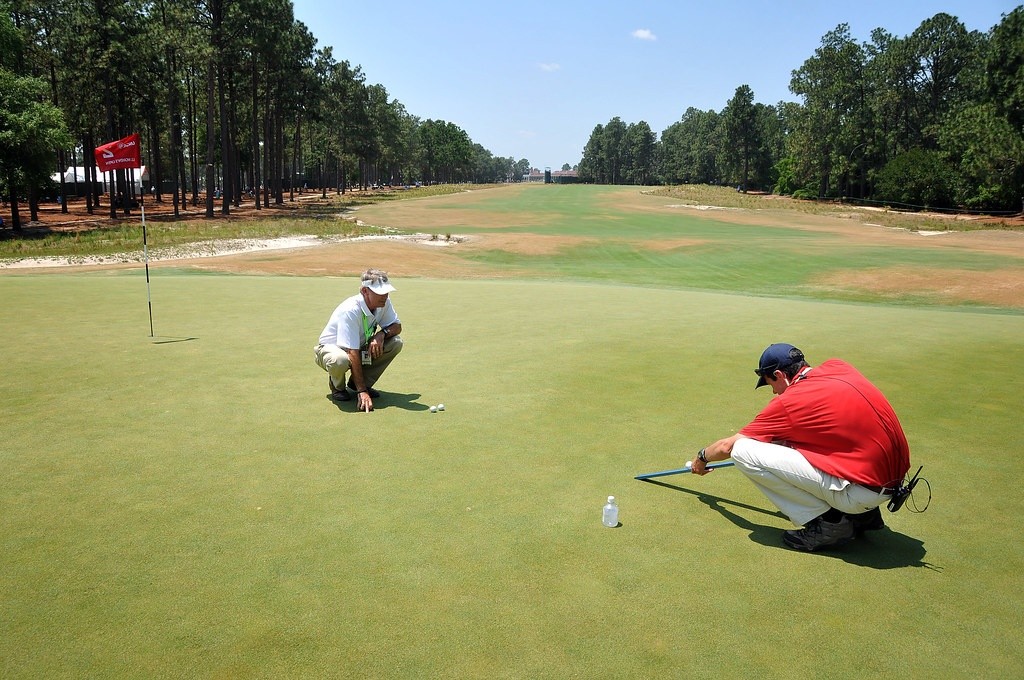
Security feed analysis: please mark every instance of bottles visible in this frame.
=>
[602,496,619,528]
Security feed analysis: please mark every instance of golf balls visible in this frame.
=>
[429,406,437,412]
[438,404,444,410]
[685,461,692,467]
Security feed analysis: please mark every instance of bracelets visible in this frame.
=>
[357,390,367,393]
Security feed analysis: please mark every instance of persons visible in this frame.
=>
[365,354,369,360]
[691,343,911,550]
[313,268,403,413]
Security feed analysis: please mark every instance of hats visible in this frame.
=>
[754,343,804,390]
[361,277,397,295]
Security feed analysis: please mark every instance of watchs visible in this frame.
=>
[381,328,388,339]
[698,448,708,463]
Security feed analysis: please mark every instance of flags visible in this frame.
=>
[94,133,141,171]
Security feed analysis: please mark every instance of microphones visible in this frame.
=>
[798,374,807,380]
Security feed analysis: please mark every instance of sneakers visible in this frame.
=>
[782,515,855,552]
[845,506,884,533]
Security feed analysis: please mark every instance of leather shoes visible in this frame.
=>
[329,376,351,401]
[347,377,380,398]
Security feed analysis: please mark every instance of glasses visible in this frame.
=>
[755,364,776,377]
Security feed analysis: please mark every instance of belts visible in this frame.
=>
[860,484,895,495]
